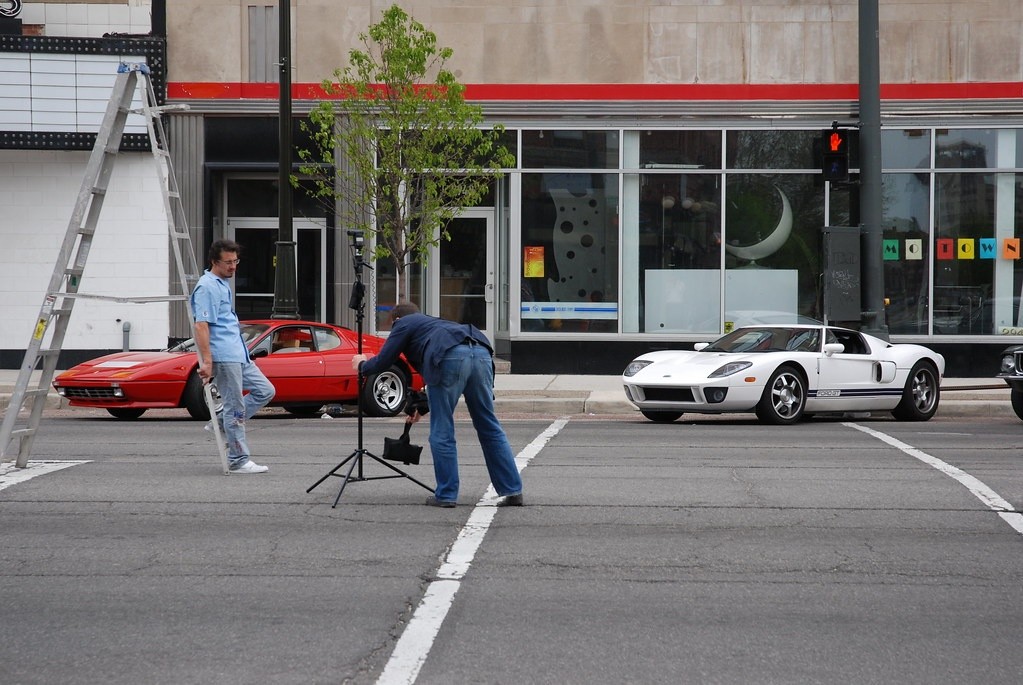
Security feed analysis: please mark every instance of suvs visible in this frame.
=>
[997,344,1023,420]
[916,297,1021,336]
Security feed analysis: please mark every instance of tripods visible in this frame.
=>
[307,253,435,508]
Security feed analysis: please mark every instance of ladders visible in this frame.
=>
[0,61,231,477]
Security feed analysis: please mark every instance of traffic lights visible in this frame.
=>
[820,130,849,181]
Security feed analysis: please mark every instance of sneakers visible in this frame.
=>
[203,416,226,438]
[230,460,268,474]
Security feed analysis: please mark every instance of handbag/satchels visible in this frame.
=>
[382,422,423,465]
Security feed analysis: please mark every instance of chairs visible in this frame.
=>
[282,339,301,349]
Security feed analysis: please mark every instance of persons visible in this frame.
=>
[350,301,523,507]
[190,240,275,474]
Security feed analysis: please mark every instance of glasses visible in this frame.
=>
[216,258,240,265]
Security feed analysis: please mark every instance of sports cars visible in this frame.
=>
[652,309,823,333]
[621,325,945,425]
[51,318,426,421]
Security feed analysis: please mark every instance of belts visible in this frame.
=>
[461,338,481,346]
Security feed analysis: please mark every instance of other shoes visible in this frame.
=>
[425,495,456,508]
[497,494,523,506]
